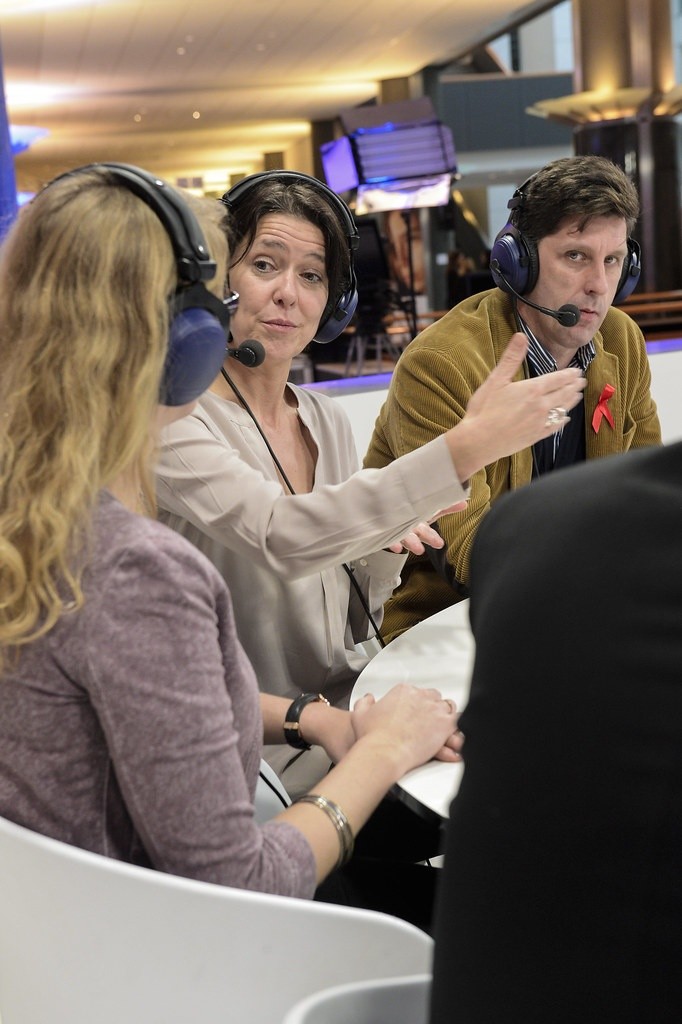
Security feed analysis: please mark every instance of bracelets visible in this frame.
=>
[283,693,331,751]
[290,794,355,864]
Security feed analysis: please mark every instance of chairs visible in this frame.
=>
[0,812,434,1024]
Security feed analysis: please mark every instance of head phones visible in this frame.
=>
[218,170,360,345]
[488,172,642,306]
[35,160,231,406]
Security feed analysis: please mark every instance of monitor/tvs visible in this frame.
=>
[317,118,459,196]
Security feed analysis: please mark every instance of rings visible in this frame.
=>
[545,408,566,427]
[444,700,455,712]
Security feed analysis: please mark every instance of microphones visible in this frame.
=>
[499,275,580,327]
[226,339,266,368]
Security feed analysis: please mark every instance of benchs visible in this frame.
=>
[299,335,682,449]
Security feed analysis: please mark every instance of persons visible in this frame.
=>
[139,170,588,814]
[365,153,663,640]
[0,162,464,897]
[432,442,681,1023]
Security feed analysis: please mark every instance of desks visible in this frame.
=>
[350,597,476,818]
